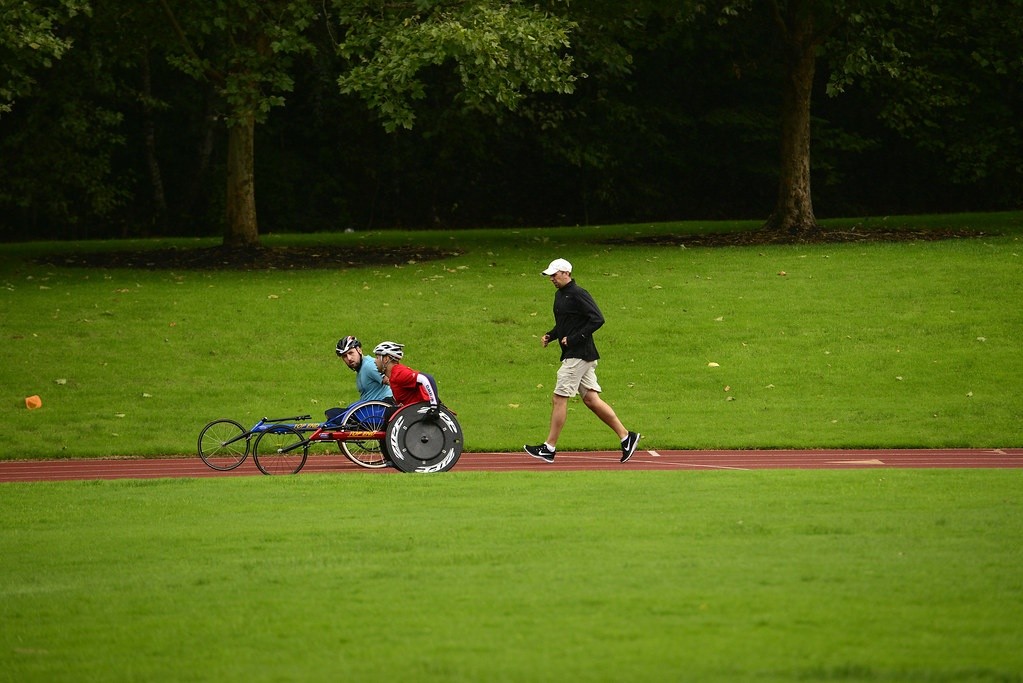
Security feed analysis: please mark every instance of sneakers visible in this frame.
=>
[523,442,556,463]
[620,431,640,463]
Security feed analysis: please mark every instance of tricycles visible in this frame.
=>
[198,373,465,475]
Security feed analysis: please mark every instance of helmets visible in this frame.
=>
[336,336,362,357]
[373,341,405,362]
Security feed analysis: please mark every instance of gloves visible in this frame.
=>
[422,408,439,423]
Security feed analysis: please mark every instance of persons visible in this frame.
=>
[325,335,393,431]
[372,341,439,464]
[524,258,640,463]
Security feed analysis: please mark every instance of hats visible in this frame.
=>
[542,258,572,275]
